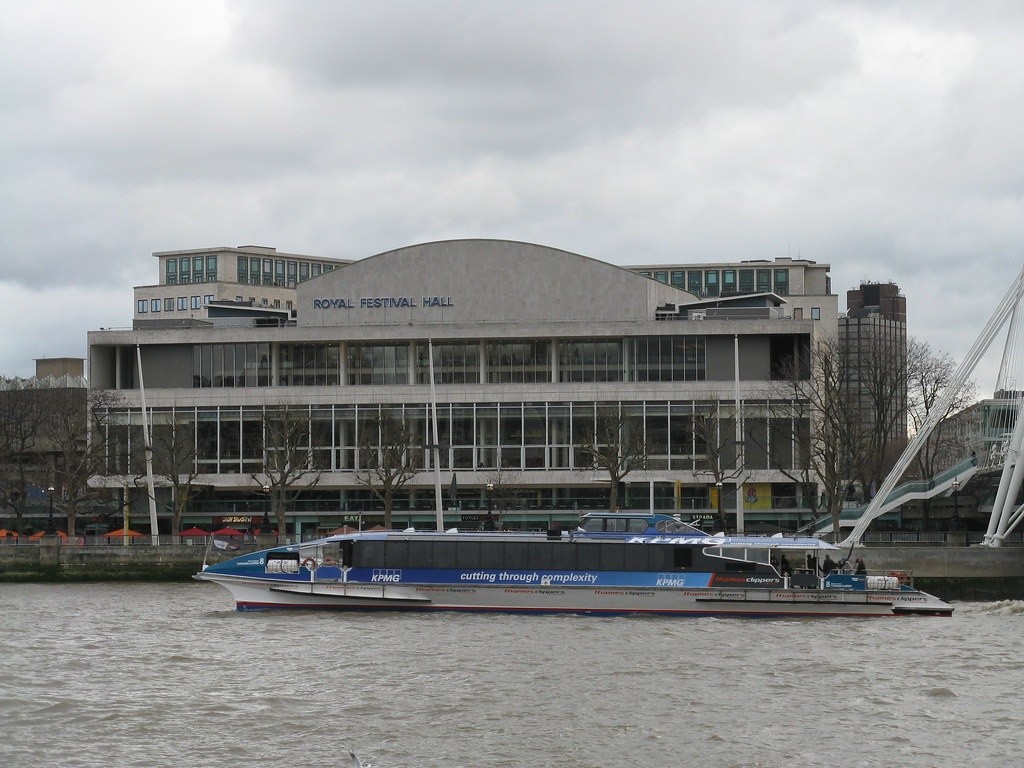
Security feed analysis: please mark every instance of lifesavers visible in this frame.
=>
[303,558,316,567]
[895,573,907,585]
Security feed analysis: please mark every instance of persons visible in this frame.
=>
[42,532,88,546]
[928,470,935,491]
[6,530,14,544]
[794,516,824,540]
[313,493,632,511]
[818,491,827,509]
[764,550,867,589]
[243,526,324,543]
[484,517,496,531]
[970,450,976,466]
[845,476,886,503]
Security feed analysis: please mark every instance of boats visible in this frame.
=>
[191,511,956,619]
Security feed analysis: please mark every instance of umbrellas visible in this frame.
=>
[0,528,69,542]
[325,523,385,535]
[102,527,145,539]
[177,526,244,536]
